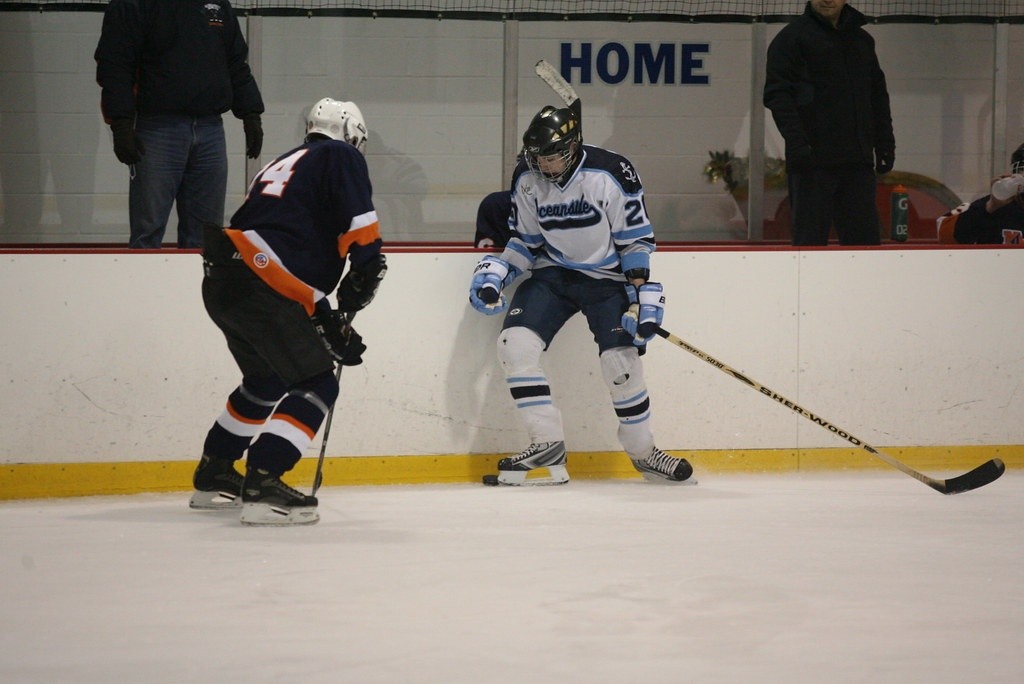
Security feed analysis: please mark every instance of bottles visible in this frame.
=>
[992,173,1024,200]
[891,184,908,241]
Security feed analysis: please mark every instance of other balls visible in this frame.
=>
[482,475,498,486]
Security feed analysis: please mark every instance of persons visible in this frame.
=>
[474,146,526,247]
[190,98,388,527]
[763,0,896,246]
[936,143,1024,244]
[93,0,265,249]
[470,107,699,485]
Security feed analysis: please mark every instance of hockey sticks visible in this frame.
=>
[534,58,583,144]
[654,323,1006,496]
[312,313,356,497]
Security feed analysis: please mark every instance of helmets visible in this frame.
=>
[522,105,582,182]
[304,98,367,153]
[1011,138,1024,208]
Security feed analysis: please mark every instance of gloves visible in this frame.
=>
[469,255,516,313]
[110,120,145,165]
[620,281,665,347]
[338,257,387,313]
[791,146,818,171]
[310,309,367,366]
[243,117,263,159]
[875,145,896,174]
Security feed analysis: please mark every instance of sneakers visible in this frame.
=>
[632,448,696,487]
[497,436,570,487]
[242,459,319,527]
[189,448,245,509]
